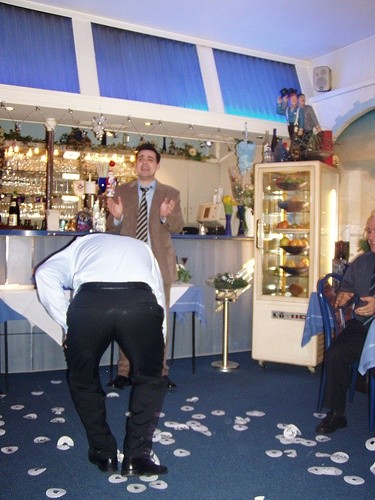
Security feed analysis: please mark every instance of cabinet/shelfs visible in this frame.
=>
[0,140,139,195]
[153,147,265,227]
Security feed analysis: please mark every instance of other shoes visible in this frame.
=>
[161,375,178,389]
[315,411,348,435]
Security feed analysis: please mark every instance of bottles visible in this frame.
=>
[290,126,302,160]
[262,128,289,161]
[8,191,19,226]
[332,240,347,287]
[19,220,46,231]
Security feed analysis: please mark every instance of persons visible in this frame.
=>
[278,88,321,151]
[105,145,184,390]
[316,209,375,433]
[35,233,167,478]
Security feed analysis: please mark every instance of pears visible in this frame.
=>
[280,237,309,246]
[277,220,304,229]
[284,258,309,267]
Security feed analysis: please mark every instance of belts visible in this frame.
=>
[78,284,152,290]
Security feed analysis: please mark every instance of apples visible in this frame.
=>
[288,283,303,295]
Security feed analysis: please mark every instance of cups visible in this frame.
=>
[0,152,143,224]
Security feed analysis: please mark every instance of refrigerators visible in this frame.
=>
[253,161,340,369]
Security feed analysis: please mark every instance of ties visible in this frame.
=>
[136,186,152,244]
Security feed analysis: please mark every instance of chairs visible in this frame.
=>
[317,273,361,412]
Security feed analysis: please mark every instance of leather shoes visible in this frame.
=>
[107,376,133,389]
[120,456,169,478]
[87,455,118,471]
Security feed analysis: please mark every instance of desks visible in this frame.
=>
[302,292,375,376]
[0,285,205,393]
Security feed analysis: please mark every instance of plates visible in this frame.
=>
[274,228,310,235]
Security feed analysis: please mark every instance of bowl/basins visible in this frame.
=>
[277,181,304,189]
[277,199,309,212]
[279,266,308,275]
[279,245,309,255]
